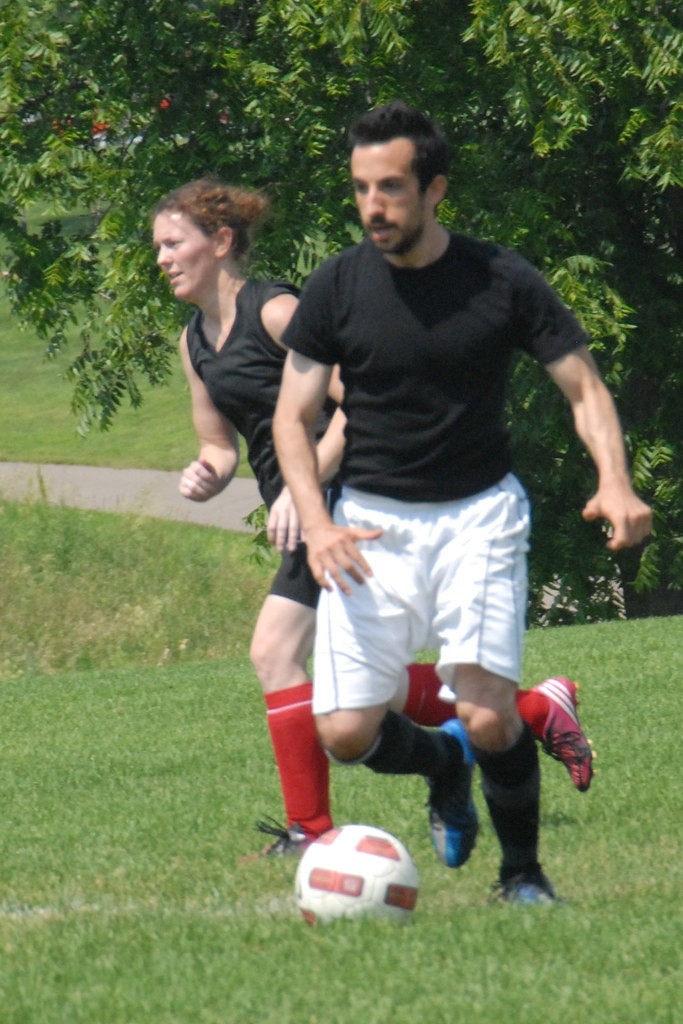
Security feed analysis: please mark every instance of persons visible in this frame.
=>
[272,106,653,907]
[149,181,593,869]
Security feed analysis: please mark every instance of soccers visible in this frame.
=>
[294,825,419,929]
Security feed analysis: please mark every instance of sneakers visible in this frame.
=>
[489,862,557,908]
[425,720,479,869]
[240,814,309,866]
[532,676,598,793]
